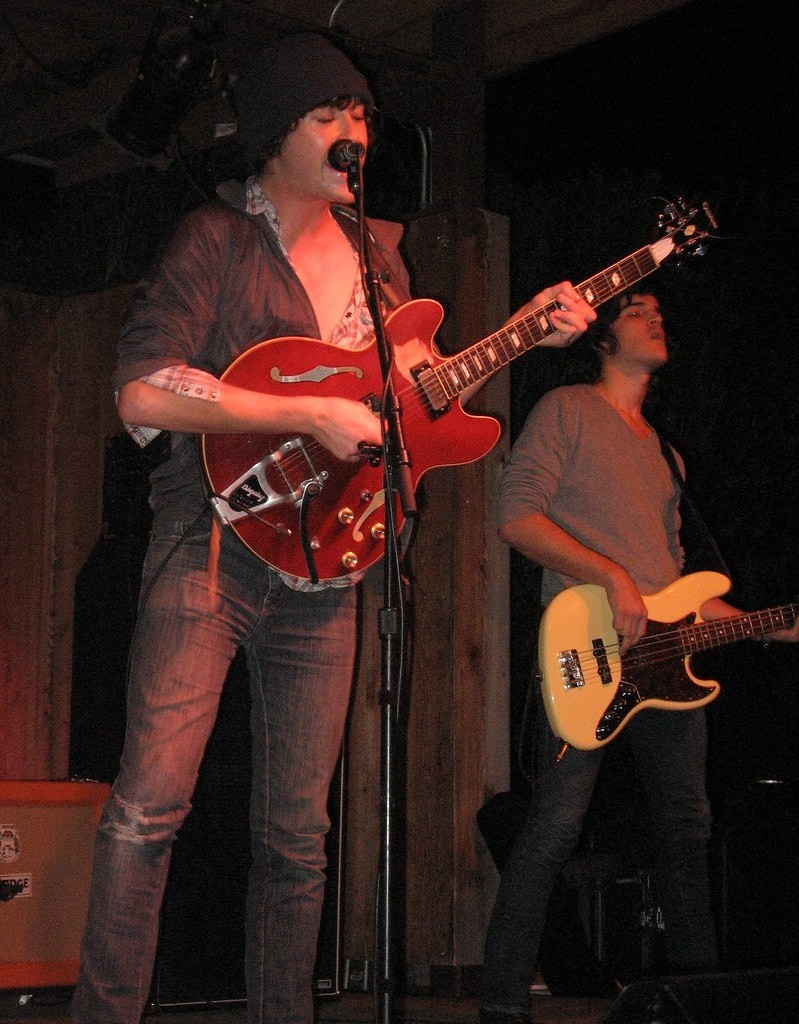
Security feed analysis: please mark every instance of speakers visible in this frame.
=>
[600,966,799,1024]
[0,647,342,1014]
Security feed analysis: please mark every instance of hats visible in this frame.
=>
[237,34,374,173]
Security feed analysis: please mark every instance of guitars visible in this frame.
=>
[535,566,799,756]
[193,194,722,591]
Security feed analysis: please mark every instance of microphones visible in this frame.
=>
[327,139,365,172]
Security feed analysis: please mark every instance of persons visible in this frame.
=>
[478,282,799,1024]
[70,30,598,1024]
